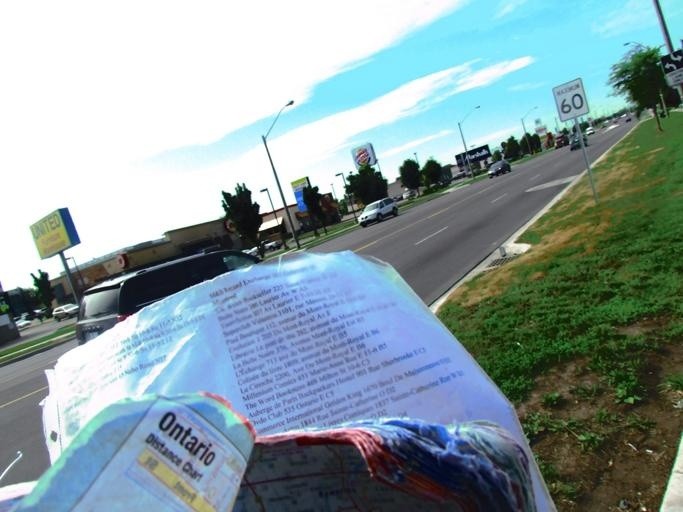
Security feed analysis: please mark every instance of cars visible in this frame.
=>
[354,197,401,227]
[486,160,514,179]
[402,190,417,199]
[242,238,280,256]
[52,303,79,322]
[571,112,631,151]
[12,308,48,331]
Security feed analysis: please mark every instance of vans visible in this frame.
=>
[78,247,263,345]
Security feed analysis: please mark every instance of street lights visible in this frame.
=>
[261,100,301,251]
[335,171,360,224]
[457,105,482,180]
[521,106,538,154]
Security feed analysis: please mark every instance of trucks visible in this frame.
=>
[553,133,568,148]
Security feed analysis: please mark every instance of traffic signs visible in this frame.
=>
[552,77,591,122]
